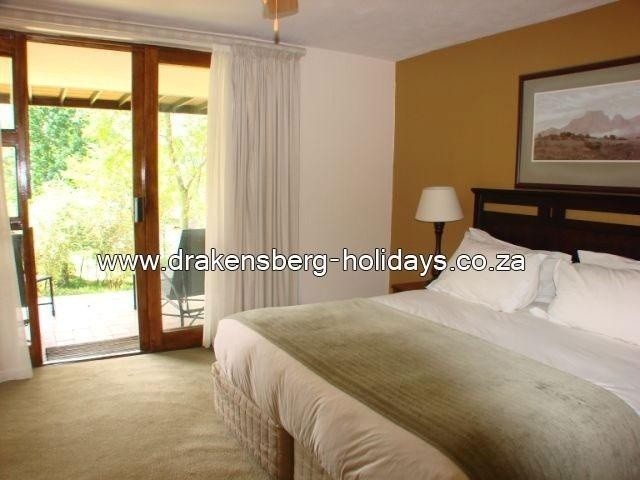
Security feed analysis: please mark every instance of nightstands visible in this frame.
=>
[391,280,428,293]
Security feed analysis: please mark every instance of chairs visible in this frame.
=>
[11,234,56,342]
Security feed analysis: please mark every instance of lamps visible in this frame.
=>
[260,0,299,46]
[414,186,465,286]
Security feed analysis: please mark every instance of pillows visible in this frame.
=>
[425,227,640,347]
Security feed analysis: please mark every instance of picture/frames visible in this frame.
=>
[514,54,640,195]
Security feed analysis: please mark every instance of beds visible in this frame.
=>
[210,187,640,480]
